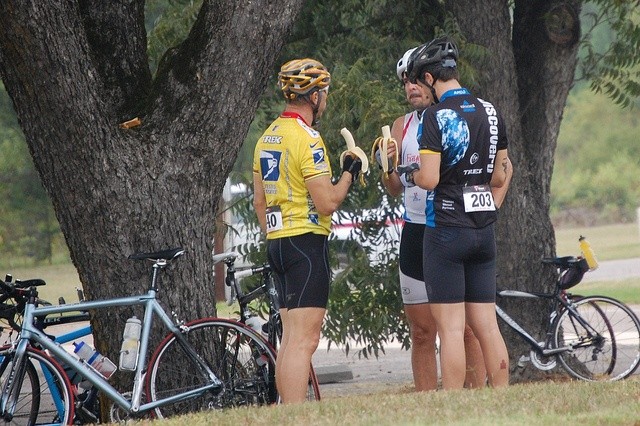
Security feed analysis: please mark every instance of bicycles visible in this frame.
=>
[565,291,617,376]
[495,256,640,385]
[212,250,322,402]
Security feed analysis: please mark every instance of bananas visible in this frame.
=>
[371,125,399,180]
[339,127,368,188]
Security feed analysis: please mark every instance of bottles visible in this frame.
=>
[69,339,115,380]
[121,316,142,370]
[578,235,599,271]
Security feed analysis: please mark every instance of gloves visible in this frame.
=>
[342,154,361,181]
[375,149,393,169]
[398,163,419,188]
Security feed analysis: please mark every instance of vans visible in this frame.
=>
[229,179,407,291]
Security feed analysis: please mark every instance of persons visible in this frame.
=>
[396,36,510,391]
[374,46,512,392]
[253,59,362,406]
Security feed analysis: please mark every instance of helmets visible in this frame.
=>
[278,59,333,98]
[396,46,416,81]
[408,37,460,82]
